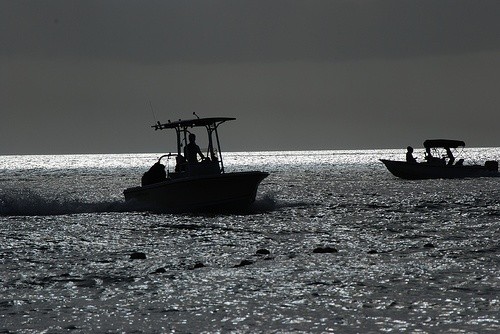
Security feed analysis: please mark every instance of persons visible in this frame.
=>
[441,143,456,165]
[182,132,206,164]
[404,146,419,165]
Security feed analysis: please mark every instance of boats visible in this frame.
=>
[378,138,498,179]
[123,112,271,214]
